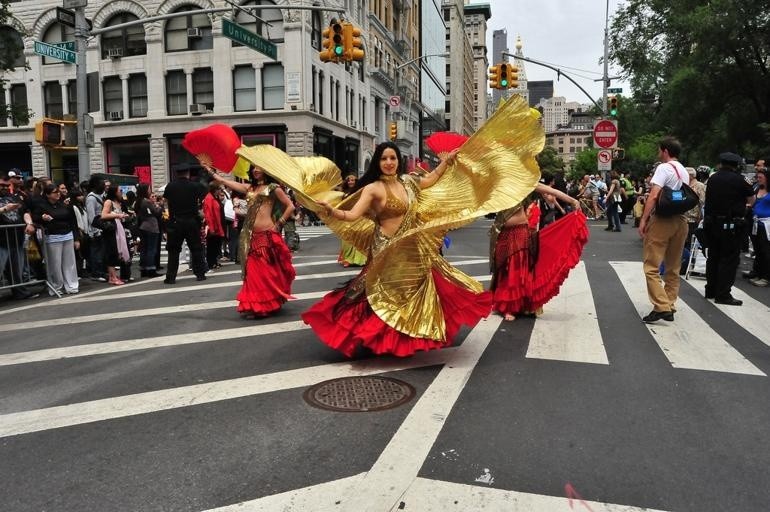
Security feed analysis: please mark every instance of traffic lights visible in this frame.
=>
[34,119,66,148]
[345,24,364,62]
[319,26,333,62]
[329,23,346,60]
[498,64,510,90]
[613,148,625,160]
[509,66,519,89]
[390,122,397,141]
[489,64,499,90]
[609,97,618,117]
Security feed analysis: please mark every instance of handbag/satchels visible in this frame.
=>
[91,199,117,232]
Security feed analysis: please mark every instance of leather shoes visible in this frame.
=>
[642,309,675,323]
[715,298,744,306]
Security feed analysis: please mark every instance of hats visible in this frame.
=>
[8,168,25,178]
[654,163,702,218]
[175,162,193,170]
[718,151,742,165]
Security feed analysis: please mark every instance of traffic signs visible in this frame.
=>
[57,6,75,29]
[49,42,75,52]
[222,18,277,62]
[34,41,76,64]
[608,88,622,93]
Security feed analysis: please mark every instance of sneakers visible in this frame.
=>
[605,219,640,233]
[749,276,759,284]
[754,278,770,288]
[163,253,241,285]
[139,269,164,278]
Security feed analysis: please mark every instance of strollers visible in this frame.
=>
[685,218,709,280]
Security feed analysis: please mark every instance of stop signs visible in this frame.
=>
[593,120,619,149]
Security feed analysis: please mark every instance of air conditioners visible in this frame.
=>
[351,120,357,127]
[109,111,122,119]
[109,48,123,57]
[311,40,318,48]
[347,66,353,74]
[187,28,203,37]
[189,104,206,114]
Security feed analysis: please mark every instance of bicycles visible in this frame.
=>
[576,195,608,220]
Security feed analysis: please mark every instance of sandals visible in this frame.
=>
[109,276,126,285]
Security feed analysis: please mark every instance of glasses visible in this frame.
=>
[15,176,25,180]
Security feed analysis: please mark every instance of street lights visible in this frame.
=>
[392,51,451,122]
